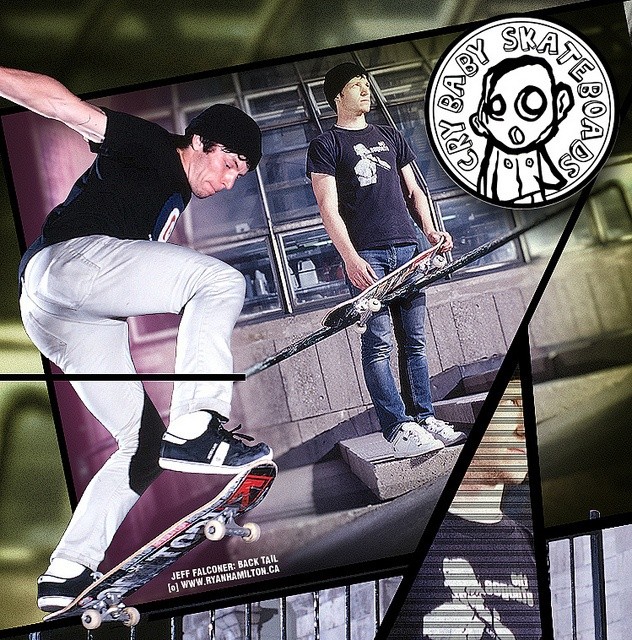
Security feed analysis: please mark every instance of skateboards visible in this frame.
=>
[320,236,449,331]
[41,461,279,630]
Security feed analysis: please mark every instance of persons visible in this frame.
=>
[306,63,466,458]
[388,368,541,639]
[1,66,273,611]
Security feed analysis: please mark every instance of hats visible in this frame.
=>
[186,103,261,165]
[322,62,367,111]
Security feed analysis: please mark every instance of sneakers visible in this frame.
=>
[158,410,274,474]
[393,423,442,457]
[422,418,468,443]
[38,562,106,612]
